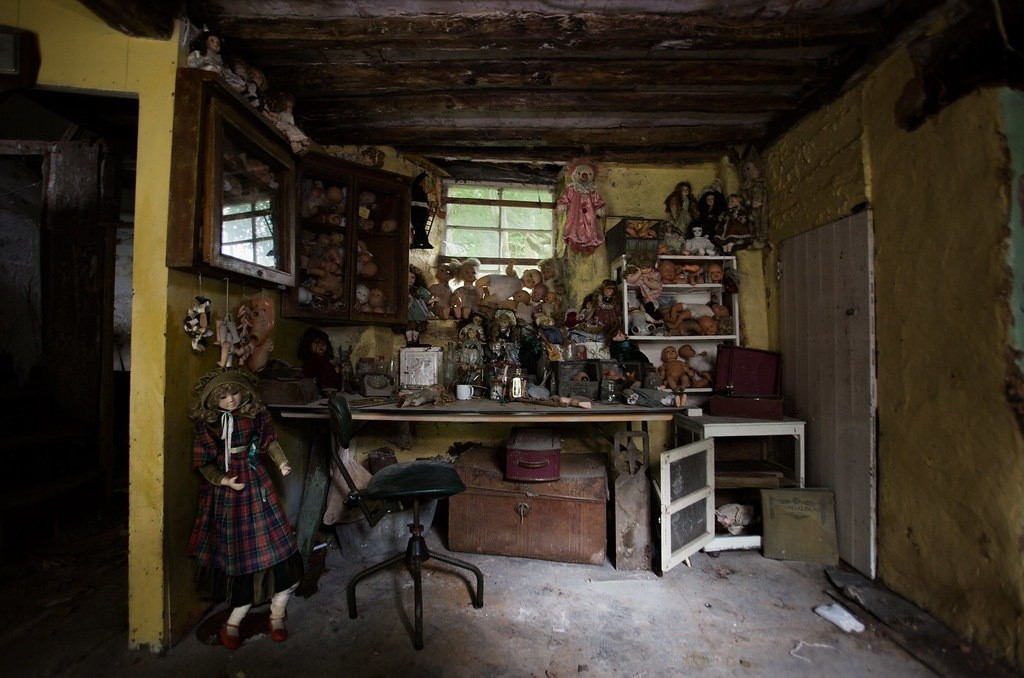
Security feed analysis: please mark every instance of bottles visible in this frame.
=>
[377,355,385,374]
[385,356,398,392]
[509,369,527,401]
[600,379,621,405]
[489,378,505,400]
[563,340,577,362]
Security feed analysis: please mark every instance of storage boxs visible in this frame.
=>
[447,441,611,568]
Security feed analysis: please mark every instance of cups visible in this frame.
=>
[456,384,474,400]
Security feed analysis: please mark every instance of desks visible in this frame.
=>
[277,386,694,580]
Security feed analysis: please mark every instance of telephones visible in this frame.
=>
[350,371,397,397]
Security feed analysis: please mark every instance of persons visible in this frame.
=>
[185,28,775,650]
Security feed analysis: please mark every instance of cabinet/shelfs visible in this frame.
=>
[174,70,300,292]
[659,414,808,574]
[276,152,414,326]
[610,252,744,395]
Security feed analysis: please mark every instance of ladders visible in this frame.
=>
[424,201,439,237]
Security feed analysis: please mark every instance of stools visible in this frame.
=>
[343,461,483,653]
